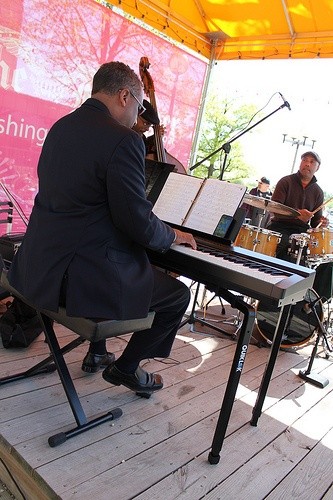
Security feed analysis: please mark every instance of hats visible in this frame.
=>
[138,99,160,125]
[301,151,321,164]
[257,177,269,184]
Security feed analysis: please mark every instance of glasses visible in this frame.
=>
[118,88,147,116]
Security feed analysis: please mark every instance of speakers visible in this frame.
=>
[0,235,24,269]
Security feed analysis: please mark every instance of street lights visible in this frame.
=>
[281,133,316,174]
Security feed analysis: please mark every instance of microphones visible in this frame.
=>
[278,92,291,110]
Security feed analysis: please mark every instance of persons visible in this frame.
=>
[7,62,191,393]
[249,176,273,228]
[133,99,165,158]
[270,152,329,265]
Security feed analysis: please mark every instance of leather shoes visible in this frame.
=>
[102,362,163,392]
[82,352,115,373]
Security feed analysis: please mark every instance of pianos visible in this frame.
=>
[134,236,317,467]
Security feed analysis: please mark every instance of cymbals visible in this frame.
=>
[243,192,301,218]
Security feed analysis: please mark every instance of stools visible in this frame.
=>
[0,271,156,449]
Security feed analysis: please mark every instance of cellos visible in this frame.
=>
[132,56,197,281]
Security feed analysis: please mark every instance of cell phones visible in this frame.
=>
[212,213,235,238]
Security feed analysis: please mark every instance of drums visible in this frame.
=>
[304,226,333,264]
[233,222,283,257]
[254,288,324,349]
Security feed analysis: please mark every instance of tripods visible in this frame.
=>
[176,101,287,339]
